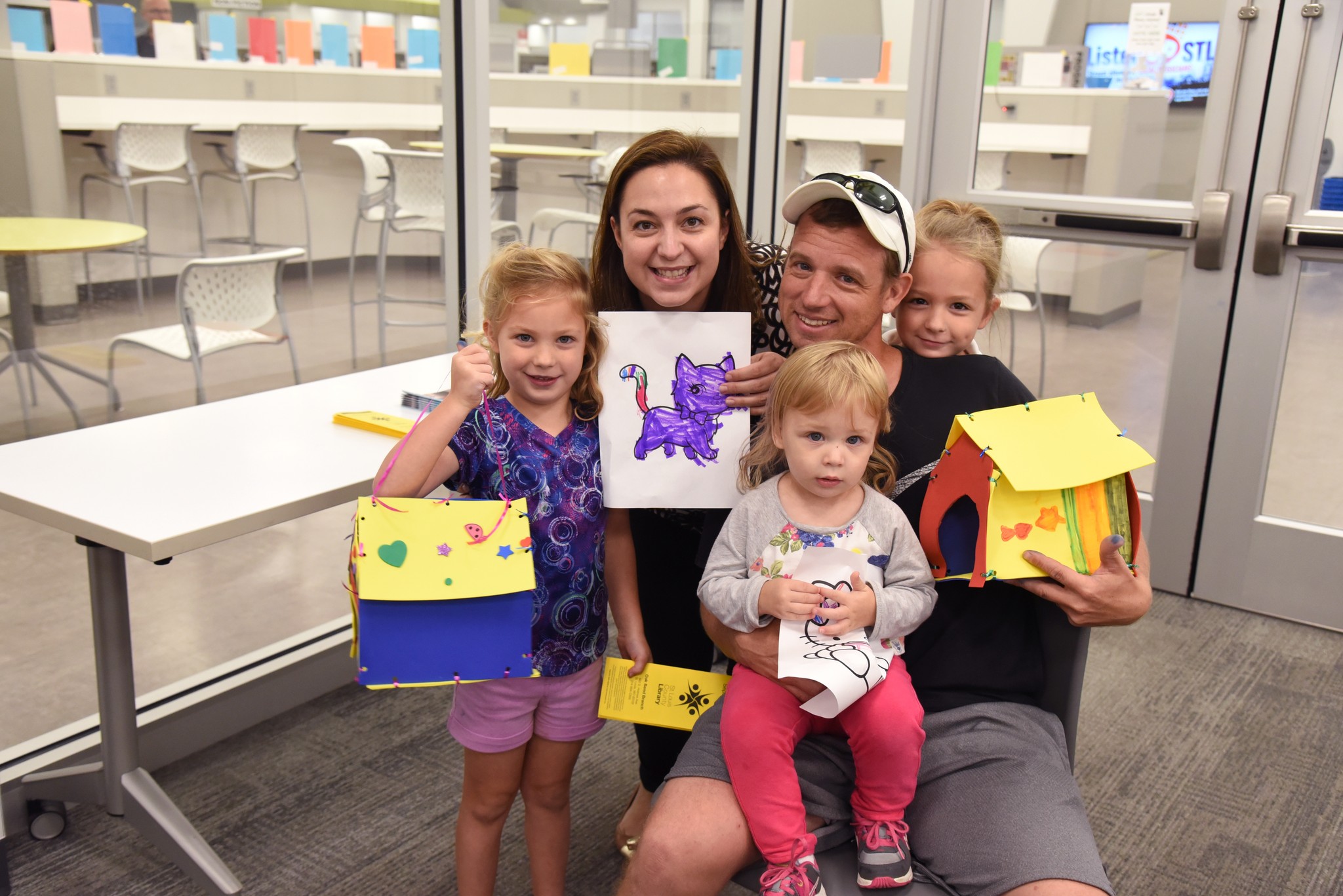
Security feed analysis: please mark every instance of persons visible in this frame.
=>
[367,246,650,896]
[578,129,800,857]
[618,173,1153,896]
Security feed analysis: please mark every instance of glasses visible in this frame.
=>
[809,172,909,274]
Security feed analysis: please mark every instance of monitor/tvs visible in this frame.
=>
[1080,20,1223,108]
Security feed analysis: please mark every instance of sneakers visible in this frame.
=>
[850,810,914,890]
[760,856,826,896]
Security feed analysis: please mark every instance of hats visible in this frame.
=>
[782,171,916,273]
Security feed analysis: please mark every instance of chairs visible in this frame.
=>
[201,122,313,284]
[107,246,305,424]
[331,137,428,370]
[526,201,612,326]
[980,237,1057,399]
[789,140,886,252]
[80,118,207,311]
[372,149,524,366]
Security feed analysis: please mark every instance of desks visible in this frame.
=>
[0,217,147,429]
[408,140,606,248]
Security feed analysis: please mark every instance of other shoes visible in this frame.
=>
[614,783,644,861]
